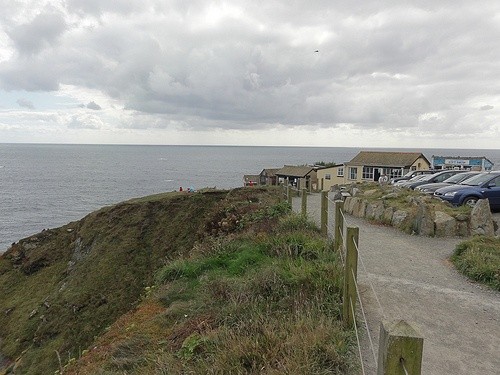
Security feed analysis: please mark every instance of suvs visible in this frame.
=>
[389,169,500,208]
